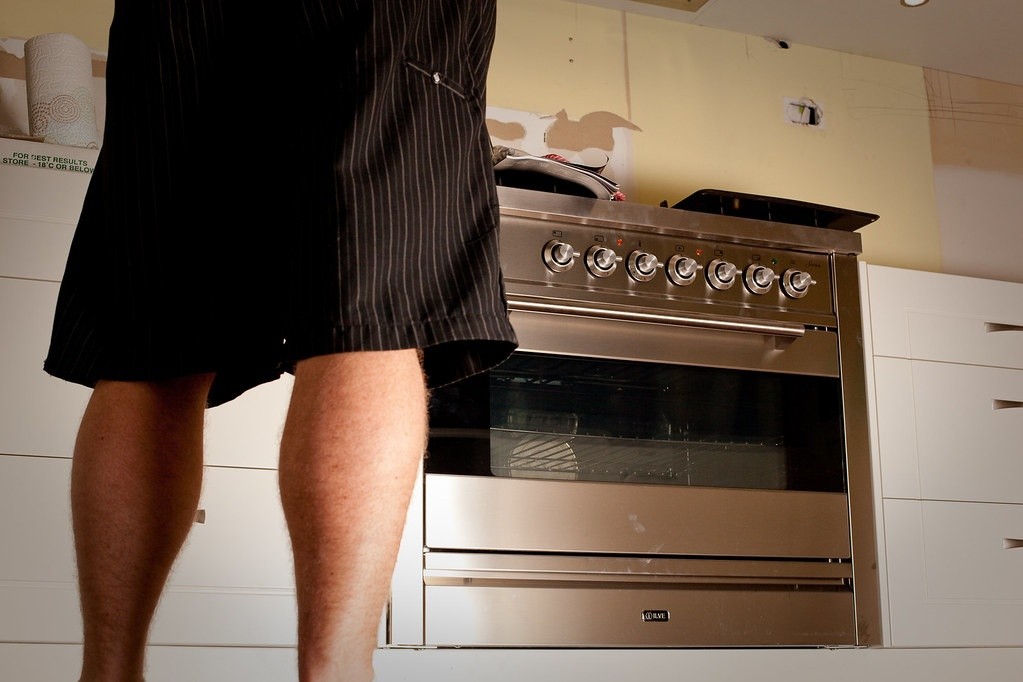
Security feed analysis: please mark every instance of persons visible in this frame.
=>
[43,0,519,682]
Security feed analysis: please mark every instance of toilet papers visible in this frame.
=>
[25,32,99,150]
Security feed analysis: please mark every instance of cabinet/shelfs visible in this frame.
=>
[0,274,386,649]
[867,263,1023,649]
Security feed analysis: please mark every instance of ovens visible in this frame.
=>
[386,215,885,648]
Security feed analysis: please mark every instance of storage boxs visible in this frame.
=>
[0,135,100,173]
